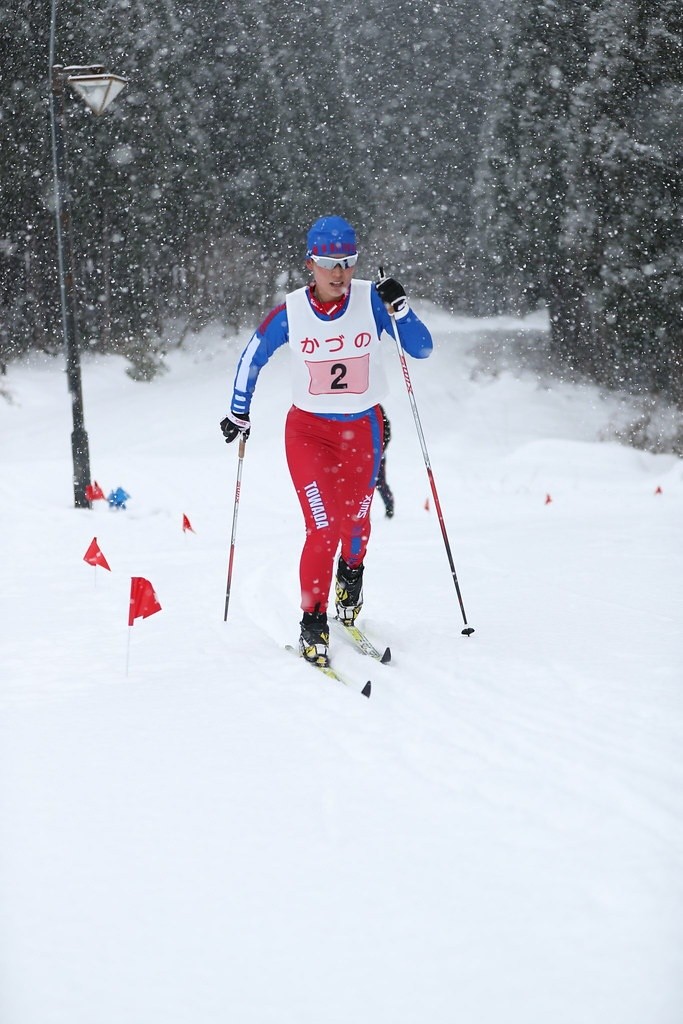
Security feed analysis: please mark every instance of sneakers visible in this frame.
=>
[336,556,364,620]
[298,611,329,662]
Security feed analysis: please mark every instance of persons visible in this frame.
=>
[220,217,433,664]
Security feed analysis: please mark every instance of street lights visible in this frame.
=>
[48,63,130,512]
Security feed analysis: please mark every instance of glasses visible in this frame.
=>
[309,251,358,270]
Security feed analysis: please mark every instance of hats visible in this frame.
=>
[306,215,357,258]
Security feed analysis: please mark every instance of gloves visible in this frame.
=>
[375,277,406,312]
[220,412,251,443]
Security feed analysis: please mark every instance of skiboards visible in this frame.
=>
[282,609,391,699]
